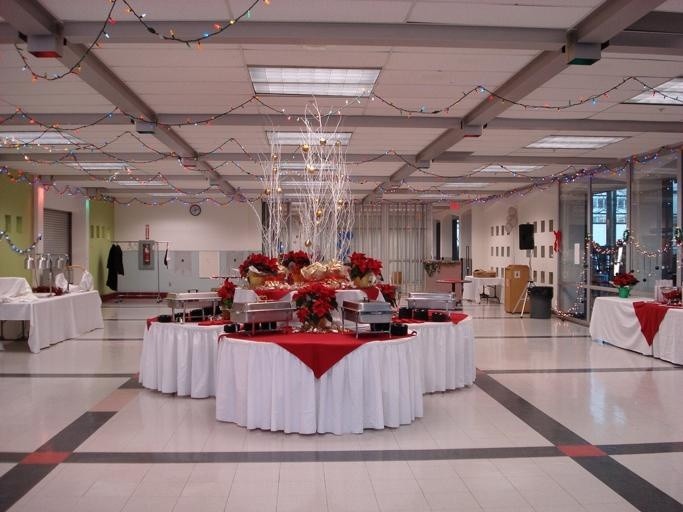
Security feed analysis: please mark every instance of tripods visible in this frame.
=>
[512,249,537,318]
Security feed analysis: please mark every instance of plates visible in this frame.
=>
[371,307,427,335]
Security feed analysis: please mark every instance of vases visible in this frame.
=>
[306,310,324,335]
[617,286,630,297]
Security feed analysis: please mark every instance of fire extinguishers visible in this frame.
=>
[143,244,150,265]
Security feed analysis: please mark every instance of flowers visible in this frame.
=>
[612,272,636,289]
[217,249,397,325]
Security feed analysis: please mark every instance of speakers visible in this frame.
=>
[519,224,534,249]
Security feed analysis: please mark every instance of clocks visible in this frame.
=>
[189,205,201,216]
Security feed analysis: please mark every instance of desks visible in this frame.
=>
[139,312,474,398]
[435,275,503,310]
[214,329,424,437]
[0,290,100,348]
[590,294,683,368]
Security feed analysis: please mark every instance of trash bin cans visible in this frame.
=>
[529,286,553,319]
[505,265,530,314]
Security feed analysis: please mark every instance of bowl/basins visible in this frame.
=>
[432,312,443,321]
